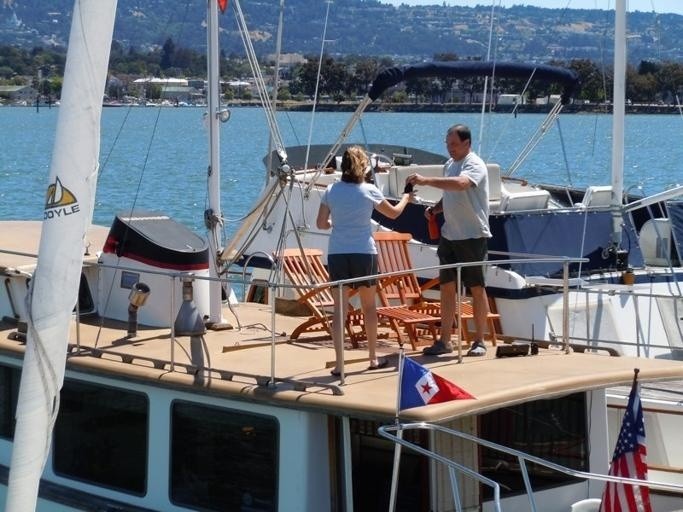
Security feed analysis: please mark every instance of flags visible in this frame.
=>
[597,382,652,512]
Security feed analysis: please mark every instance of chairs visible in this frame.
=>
[271,231,501,351]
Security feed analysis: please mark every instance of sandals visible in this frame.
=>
[423,340,453,355]
[467,340,487,355]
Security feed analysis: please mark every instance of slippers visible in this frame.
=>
[369,357,386,370]
[330,368,342,376]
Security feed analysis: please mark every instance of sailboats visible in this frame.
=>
[0,0,683,512]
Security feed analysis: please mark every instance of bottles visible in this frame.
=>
[623,267,635,285]
[428,210,440,240]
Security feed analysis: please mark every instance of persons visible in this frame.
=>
[405,125,494,355]
[315,145,419,377]
